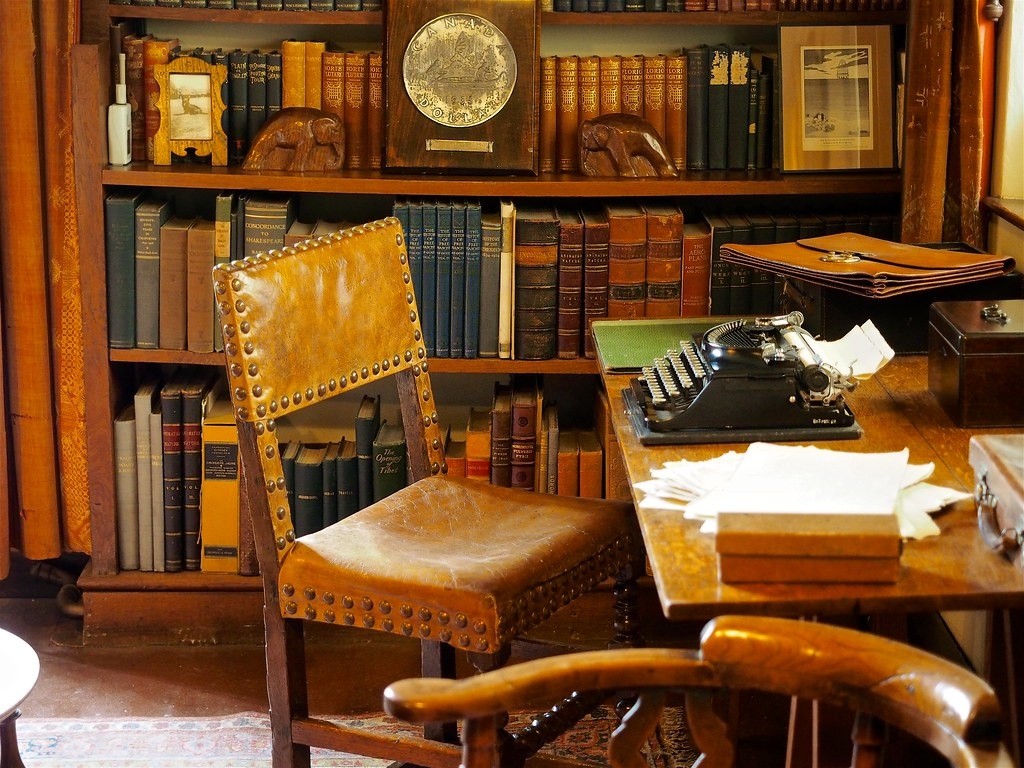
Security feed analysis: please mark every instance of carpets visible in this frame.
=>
[14,694,692,768]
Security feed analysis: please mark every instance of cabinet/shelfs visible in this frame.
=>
[75,0,913,651]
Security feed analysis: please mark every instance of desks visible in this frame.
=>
[589,280,1024,618]
[0,625,52,768]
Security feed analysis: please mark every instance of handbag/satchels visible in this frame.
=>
[720,231,1017,300]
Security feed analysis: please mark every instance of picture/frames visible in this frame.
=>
[777,19,898,174]
[154,54,244,168]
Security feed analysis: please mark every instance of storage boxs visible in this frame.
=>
[713,510,904,585]
[927,300,1024,428]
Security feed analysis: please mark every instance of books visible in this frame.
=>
[103,189,1018,360]
[109,1,907,177]
[587,314,778,374]
[715,514,902,584]
[112,365,604,574]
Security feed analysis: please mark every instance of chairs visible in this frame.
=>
[383,614,1024,768]
[210,213,638,768]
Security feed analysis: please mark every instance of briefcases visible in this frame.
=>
[968,434,1024,575]
[927,299,1024,427]
